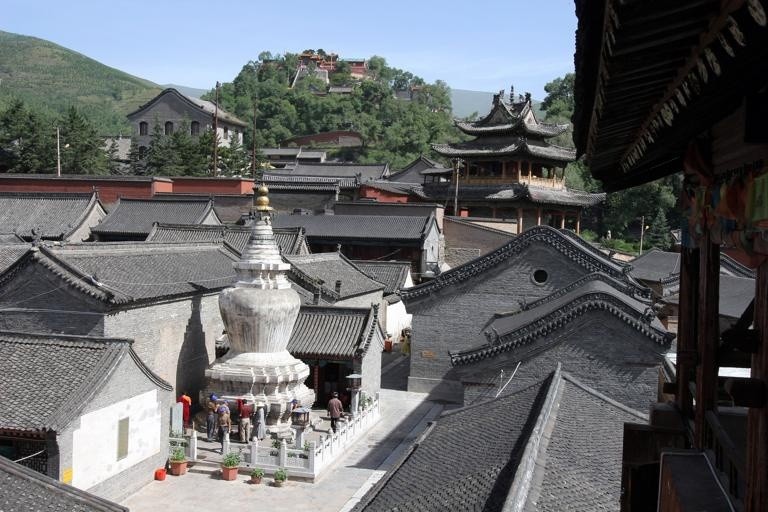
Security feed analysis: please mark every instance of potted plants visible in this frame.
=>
[272,469,289,487]
[219,451,243,482]
[168,443,189,477]
[250,466,265,485]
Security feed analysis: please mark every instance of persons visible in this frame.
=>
[219,399,231,416]
[289,399,300,417]
[253,402,265,440]
[238,399,253,443]
[216,405,232,454]
[326,391,344,433]
[206,393,218,442]
[177,390,191,428]
